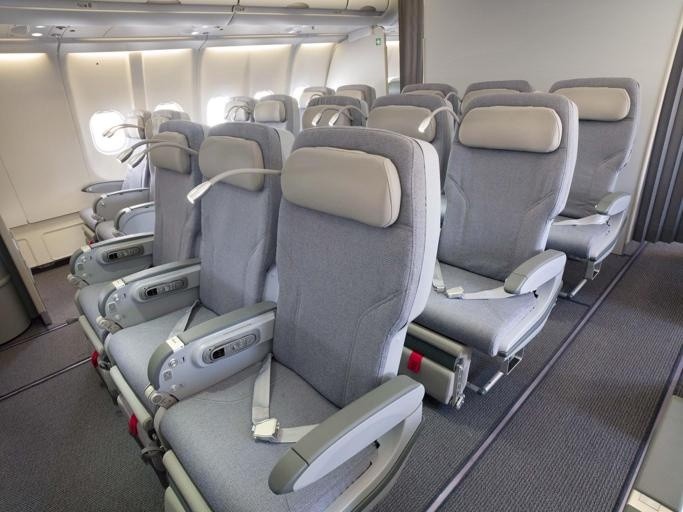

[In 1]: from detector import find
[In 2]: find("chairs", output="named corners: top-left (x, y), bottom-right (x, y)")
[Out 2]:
top-left (220, 77), bottom-right (579, 412)
top-left (68, 107), bottom-right (441, 511)
top-left (544, 77), bottom-right (644, 302)
